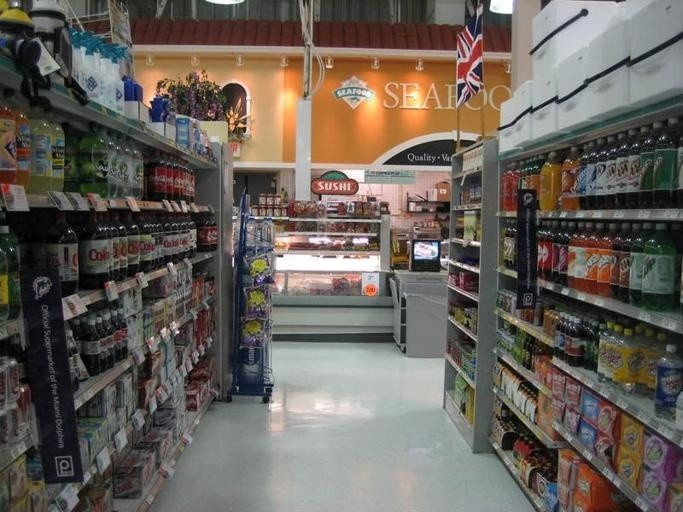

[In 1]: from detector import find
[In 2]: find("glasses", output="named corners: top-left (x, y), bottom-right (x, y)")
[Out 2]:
top-left (141, 52), bottom-right (513, 76)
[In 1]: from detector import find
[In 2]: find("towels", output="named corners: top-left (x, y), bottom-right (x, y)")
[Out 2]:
top-left (403, 279), bottom-right (447, 357)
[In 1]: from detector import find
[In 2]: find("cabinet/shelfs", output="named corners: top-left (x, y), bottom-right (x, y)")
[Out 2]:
top-left (423, 217), bottom-right (439, 228)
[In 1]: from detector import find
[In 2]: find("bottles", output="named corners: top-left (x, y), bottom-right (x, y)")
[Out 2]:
top-left (423, 217), bottom-right (439, 228)
top-left (441, 117), bottom-right (682, 497)
top-left (0, 103), bottom-right (275, 383)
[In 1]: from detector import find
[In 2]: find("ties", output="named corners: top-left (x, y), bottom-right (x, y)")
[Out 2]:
top-left (412, 239), bottom-right (440, 271)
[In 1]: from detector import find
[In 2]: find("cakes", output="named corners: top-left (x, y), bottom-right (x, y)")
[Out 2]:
top-left (0, 355), bottom-right (32, 448)
top-left (496, 286), bottom-right (596, 338)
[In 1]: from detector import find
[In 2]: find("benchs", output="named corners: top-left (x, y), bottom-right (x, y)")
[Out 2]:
top-left (455, 3), bottom-right (485, 107)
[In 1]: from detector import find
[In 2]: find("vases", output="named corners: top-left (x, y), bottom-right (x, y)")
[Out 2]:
top-left (153, 66), bottom-right (227, 124)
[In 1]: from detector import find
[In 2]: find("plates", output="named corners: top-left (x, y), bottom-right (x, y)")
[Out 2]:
top-left (489, 93), bottom-right (683, 511)
top-left (1, 49), bottom-right (221, 512)
top-left (233, 213), bottom-right (381, 298)
top-left (438, 134), bottom-right (496, 455)
